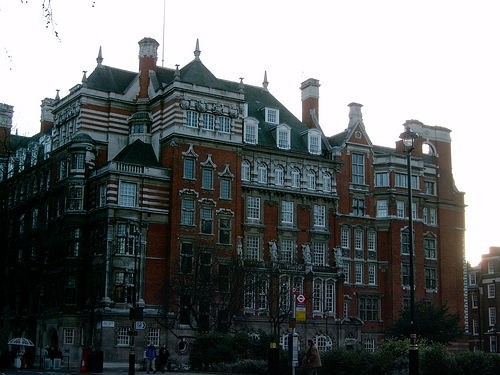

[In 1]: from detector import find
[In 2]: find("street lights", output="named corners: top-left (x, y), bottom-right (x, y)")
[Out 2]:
top-left (399, 127), bottom-right (418, 375)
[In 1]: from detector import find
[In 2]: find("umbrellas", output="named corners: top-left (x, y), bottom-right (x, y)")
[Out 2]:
top-left (7, 336), bottom-right (35, 352)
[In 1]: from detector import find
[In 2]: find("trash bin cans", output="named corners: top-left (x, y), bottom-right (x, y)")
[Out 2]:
top-left (88, 350), bottom-right (104, 373)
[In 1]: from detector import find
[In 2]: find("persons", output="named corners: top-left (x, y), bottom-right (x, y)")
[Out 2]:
top-left (42, 344), bottom-right (63, 360)
top-left (144, 340), bottom-right (157, 374)
top-left (303, 339), bottom-right (322, 375)
top-left (16, 345), bottom-right (26, 370)
top-left (158, 343), bottom-right (170, 374)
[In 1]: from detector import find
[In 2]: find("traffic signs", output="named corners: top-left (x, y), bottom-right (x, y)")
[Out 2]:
top-left (136, 321), bottom-right (147, 330)
